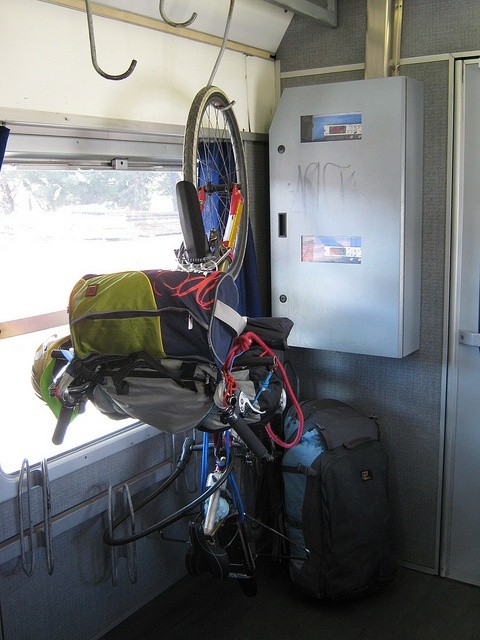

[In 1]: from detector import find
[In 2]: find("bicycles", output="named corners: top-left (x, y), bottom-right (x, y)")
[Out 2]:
top-left (104, 85), bottom-right (311, 595)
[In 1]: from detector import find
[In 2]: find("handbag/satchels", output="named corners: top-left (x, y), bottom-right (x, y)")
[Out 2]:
top-left (68, 270), bottom-right (248, 371)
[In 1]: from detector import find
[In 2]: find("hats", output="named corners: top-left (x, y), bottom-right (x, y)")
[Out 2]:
top-left (30, 332), bottom-right (78, 423)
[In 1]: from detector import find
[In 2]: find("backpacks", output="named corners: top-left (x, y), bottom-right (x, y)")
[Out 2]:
top-left (276, 397), bottom-right (404, 608)
